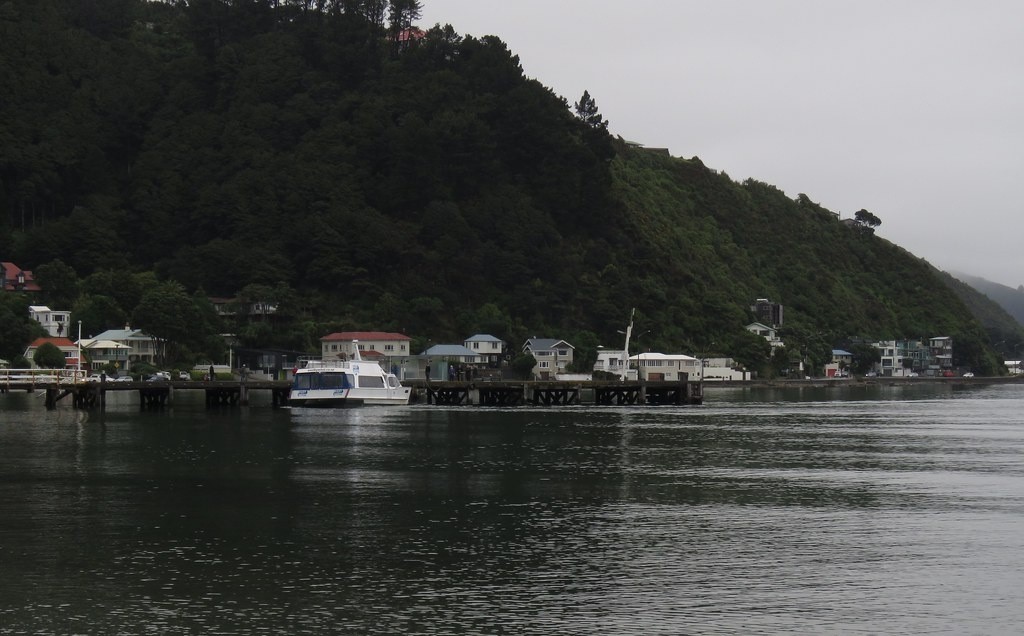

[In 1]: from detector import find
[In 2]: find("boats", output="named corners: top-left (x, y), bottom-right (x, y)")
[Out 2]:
top-left (286, 338), bottom-right (413, 409)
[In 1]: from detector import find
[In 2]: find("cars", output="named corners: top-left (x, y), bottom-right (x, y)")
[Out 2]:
top-left (88, 370), bottom-right (191, 383)
top-left (962, 372), bottom-right (975, 379)
top-left (943, 371), bottom-right (955, 377)
top-left (865, 371), bottom-right (882, 377)
top-left (833, 370), bottom-right (849, 377)
top-left (907, 371), bottom-right (919, 378)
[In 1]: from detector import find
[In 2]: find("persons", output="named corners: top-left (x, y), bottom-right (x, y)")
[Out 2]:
top-left (457, 364), bottom-right (463, 381)
top-left (465, 364), bottom-right (471, 382)
top-left (209, 362), bottom-right (215, 381)
top-left (473, 364), bottom-right (478, 378)
top-left (292, 364), bottom-right (299, 376)
top-left (449, 364), bottom-right (456, 382)
top-left (239, 363), bottom-right (247, 382)
top-left (424, 361), bottom-right (432, 380)
